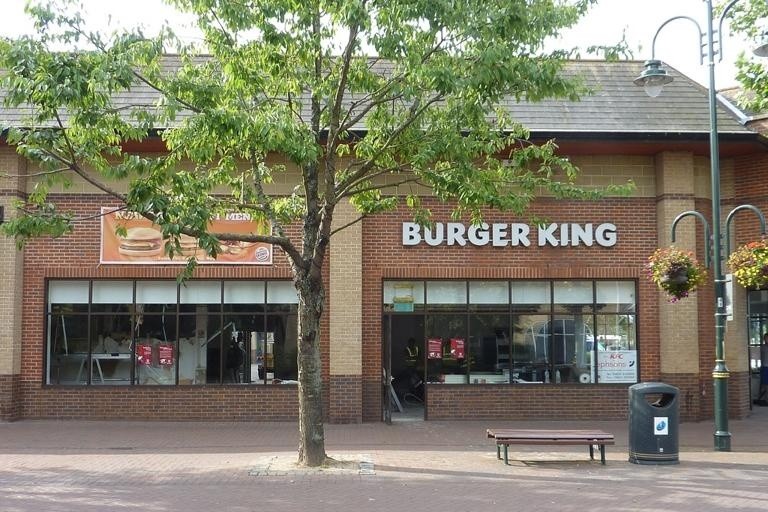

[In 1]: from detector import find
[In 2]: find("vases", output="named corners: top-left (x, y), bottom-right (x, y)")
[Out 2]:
top-left (660, 262), bottom-right (688, 286)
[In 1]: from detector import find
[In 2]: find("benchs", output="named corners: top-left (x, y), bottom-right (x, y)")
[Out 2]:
top-left (485, 429), bottom-right (616, 466)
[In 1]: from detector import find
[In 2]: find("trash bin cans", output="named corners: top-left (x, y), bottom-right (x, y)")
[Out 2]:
top-left (628, 381), bottom-right (680, 465)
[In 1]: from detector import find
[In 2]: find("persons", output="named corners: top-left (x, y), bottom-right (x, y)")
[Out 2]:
top-left (393, 337), bottom-right (420, 381)
top-left (226, 333), bottom-right (244, 383)
top-left (760, 333), bottom-right (768, 385)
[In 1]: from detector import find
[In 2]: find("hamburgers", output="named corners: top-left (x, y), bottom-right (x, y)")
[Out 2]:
top-left (118, 227), bottom-right (162, 256)
top-left (170, 224), bottom-right (202, 256)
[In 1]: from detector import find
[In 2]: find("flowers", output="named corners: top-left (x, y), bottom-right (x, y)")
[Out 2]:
top-left (725, 237), bottom-right (768, 289)
top-left (640, 246), bottom-right (710, 304)
